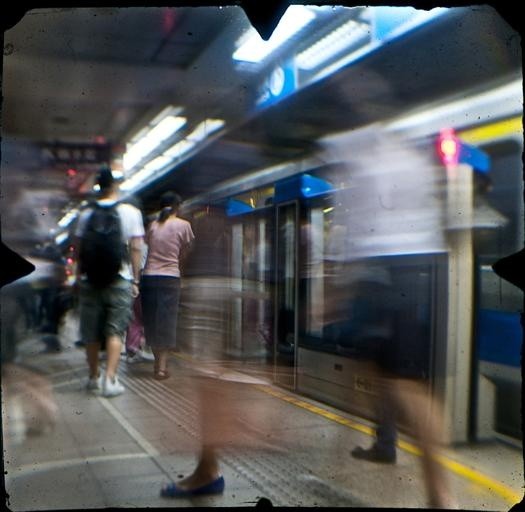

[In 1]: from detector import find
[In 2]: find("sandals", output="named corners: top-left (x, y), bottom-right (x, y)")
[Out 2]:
top-left (155, 367), bottom-right (168, 379)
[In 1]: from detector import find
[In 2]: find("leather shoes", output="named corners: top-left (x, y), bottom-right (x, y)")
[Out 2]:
top-left (162, 476), bottom-right (224, 497)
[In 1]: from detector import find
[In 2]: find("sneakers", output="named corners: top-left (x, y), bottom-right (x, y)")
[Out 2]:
top-left (85, 374), bottom-right (124, 396)
top-left (352, 443), bottom-right (395, 463)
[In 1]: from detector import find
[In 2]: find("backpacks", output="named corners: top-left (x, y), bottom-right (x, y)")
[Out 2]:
top-left (80, 199), bottom-right (125, 289)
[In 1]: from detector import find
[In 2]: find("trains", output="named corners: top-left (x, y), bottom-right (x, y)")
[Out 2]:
top-left (59, 77), bottom-right (523, 458)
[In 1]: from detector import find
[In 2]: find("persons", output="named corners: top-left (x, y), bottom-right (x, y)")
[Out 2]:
top-left (328, 65), bottom-right (460, 510)
top-left (69, 167), bottom-right (146, 398)
top-left (352, 398), bottom-right (398, 465)
top-left (162, 376), bottom-right (226, 499)
top-left (18, 191), bottom-right (228, 379)
